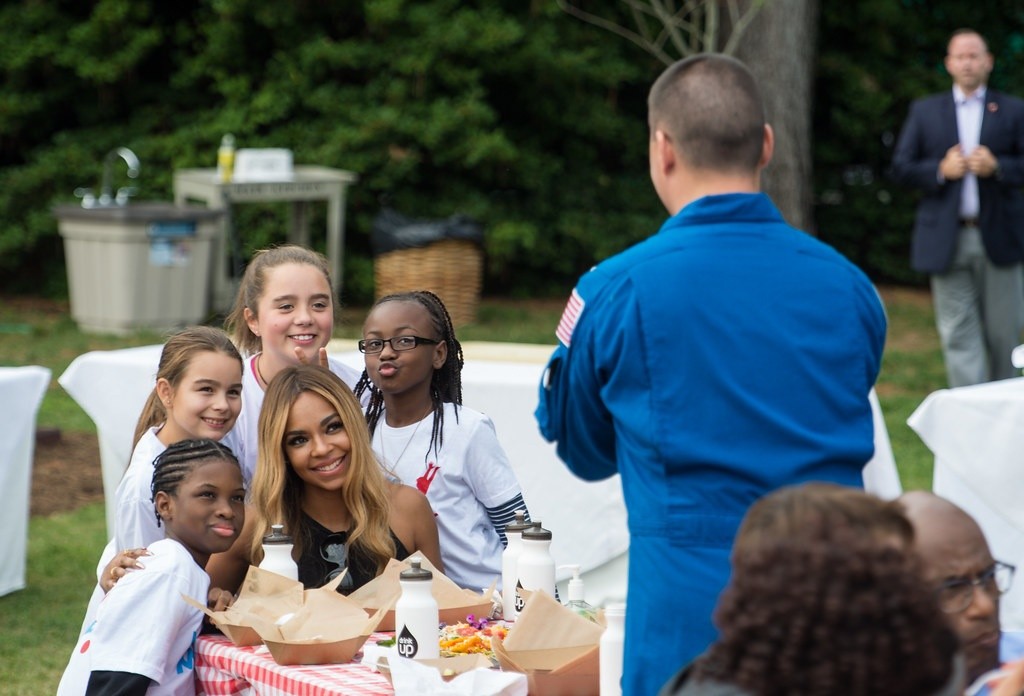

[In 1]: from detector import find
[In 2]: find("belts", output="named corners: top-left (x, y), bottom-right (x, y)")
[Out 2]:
top-left (956, 217), bottom-right (979, 229)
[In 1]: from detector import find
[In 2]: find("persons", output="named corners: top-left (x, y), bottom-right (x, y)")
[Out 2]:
top-left (661, 482), bottom-right (960, 696)
top-left (222, 241), bottom-right (384, 490)
top-left (56, 438), bottom-right (245, 696)
top-left (887, 490), bottom-right (1024, 696)
top-left (294, 290), bottom-right (561, 604)
top-left (534, 54), bottom-right (888, 696)
top-left (892, 28), bottom-right (1024, 388)
top-left (99, 363), bottom-right (446, 623)
top-left (116, 326), bottom-right (252, 554)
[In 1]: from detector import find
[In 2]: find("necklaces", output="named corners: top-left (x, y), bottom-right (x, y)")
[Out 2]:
top-left (257, 354), bottom-right (269, 388)
top-left (380, 403), bottom-right (433, 472)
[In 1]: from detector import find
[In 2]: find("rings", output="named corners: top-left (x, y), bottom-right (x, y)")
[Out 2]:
top-left (111, 567), bottom-right (119, 579)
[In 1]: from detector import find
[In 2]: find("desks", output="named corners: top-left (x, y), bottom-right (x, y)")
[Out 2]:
top-left (908, 375), bottom-right (1024, 641)
top-left (55, 336), bottom-right (904, 607)
top-left (172, 163), bottom-right (355, 319)
top-left (190, 628), bottom-right (506, 696)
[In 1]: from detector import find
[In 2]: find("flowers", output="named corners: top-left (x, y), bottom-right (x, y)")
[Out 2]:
top-left (435, 611), bottom-right (511, 657)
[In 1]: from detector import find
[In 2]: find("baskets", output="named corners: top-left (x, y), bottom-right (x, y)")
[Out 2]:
top-left (373, 235), bottom-right (485, 336)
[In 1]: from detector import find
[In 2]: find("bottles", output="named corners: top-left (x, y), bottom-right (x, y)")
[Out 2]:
top-left (514, 521), bottom-right (557, 621)
top-left (395, 556), bottom-right (440, 660)
top-left (502, 510), bottom-right (533, 622)
top-left (216, 134), bottom-right (234, 182)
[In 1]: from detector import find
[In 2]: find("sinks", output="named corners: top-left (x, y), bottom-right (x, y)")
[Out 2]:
top-left (54, 200), bottom-right (229, 239)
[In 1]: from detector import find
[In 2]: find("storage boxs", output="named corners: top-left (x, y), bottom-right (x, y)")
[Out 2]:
top-left (210, 598), bottom-right (495, 668)
top-left (375, 652), bottom-right (495, 688)
top-left (50, 202), bottom-right (226, 340)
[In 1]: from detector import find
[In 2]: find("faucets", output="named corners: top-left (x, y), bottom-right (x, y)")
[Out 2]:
top-left (73, 145), bottom-right (141, 210)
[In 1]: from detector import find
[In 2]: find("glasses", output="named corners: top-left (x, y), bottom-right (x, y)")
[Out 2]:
top-left (931, 561), bottom-right (1014, 614)
top-left (359, 335), bottom-right (440, 354)
top-left (321, 531), bottom-right (353, 594)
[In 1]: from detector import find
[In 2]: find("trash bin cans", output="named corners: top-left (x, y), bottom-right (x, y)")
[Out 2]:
top-left (373, 217), bottom-right (483, 331)
